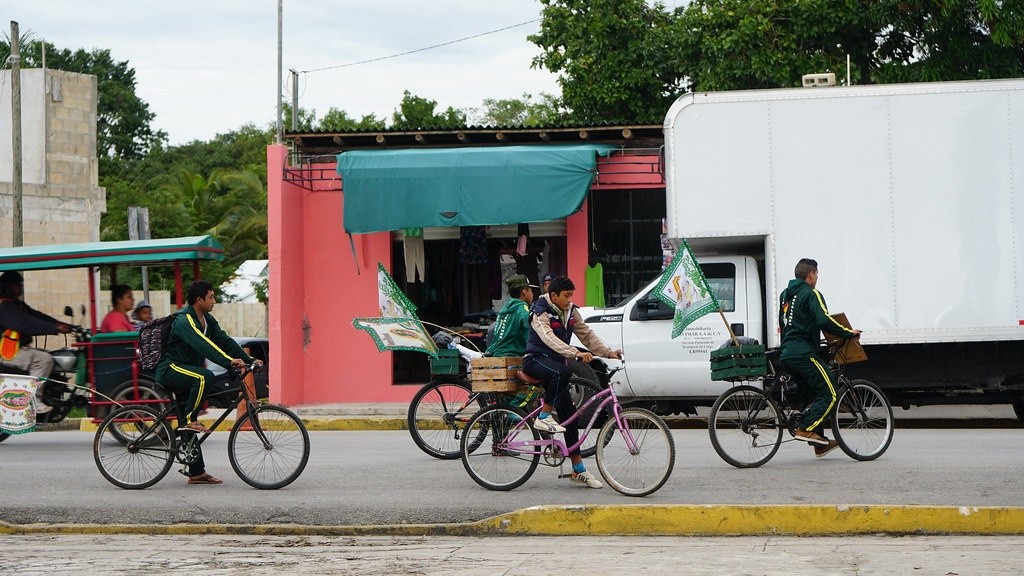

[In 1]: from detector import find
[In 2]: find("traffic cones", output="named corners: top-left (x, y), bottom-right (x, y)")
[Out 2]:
top-left (224, 346), bottom-right (268, 431)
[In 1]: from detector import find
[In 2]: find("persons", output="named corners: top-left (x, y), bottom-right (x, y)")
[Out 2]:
top-left (522, 276), bottom-right (624, 488)
top-left (543, 272), bottom-right (559, 293)
top-left (155, 282), bottom-right (263, 484)
top-left (100, 284), bottom-right (138, 333)
top-left (779, 258), bottom-right (861, 458)
top-left (0, 271), bottom-right (74, 414)
top-left (484, 275), bottom-right (545, 457)
top-left (132, 300), bottom-right (152, 330)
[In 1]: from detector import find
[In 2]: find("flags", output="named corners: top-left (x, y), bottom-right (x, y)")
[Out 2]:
top-left (352, 318), bottom-right (439, 363)
top-left (378, 261), bottom-right (418, 319)
top-left (652, 242), bottom-right (720, 340)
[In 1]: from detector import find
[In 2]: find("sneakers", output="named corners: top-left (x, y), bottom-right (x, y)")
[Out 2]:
top-left (795, 427), bottom-right (829, 445)
top-left (572, 468), bottom-right (602, 488)
top-left (534, 415), bottom-right (566, 432)
top-left (814, 439), bottom-right (839, 457)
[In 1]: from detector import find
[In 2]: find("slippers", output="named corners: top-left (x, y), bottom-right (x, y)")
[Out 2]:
top-left (187, 472), bottom-right (221, 485)
top-left (178, 419), bottom-right (210, 433)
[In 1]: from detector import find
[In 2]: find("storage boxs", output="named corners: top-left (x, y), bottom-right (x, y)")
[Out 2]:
top-left (470, 355), bottom-right (523, 393)
top-left (710, 345), bottom-right (768, 381)
top-left (429, 348), bottom-right (460, 376)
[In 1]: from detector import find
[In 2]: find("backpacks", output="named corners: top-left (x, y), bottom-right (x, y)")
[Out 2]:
top-left (138, 311), bottom-right (177, 371)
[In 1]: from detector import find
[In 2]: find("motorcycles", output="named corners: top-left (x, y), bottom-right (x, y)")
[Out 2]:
top-left (0, 233), bottom-right (225, 449)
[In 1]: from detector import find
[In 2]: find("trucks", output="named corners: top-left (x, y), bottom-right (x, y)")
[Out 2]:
top-left (548, 76), bottom-right (1024, 428)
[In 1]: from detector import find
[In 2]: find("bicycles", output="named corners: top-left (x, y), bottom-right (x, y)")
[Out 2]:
top-left (93, 363), bottom-right (310, 490)
top-left (408, 346), bottom-right (619, 461)
top-left (457, 353), bottom-right (678, 498)
top-left (706, 329), bottom-right (895, 470)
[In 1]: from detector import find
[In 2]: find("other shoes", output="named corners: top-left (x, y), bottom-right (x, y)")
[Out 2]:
top-left (35, 397), bottom-right (54, 414)
top-left (492, 444), bottom-right (521, 456)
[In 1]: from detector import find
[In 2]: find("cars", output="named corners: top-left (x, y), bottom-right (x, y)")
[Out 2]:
top-left (205, 334), bottom-right (269, 409)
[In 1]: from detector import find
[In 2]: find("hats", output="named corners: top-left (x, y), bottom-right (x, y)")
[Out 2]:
top-left (132, 302), bottom-right (154, 320)
top-left (505, 274), bottom-right (540, 289)
top-left (543, 273), bottom-right (557, 281)
